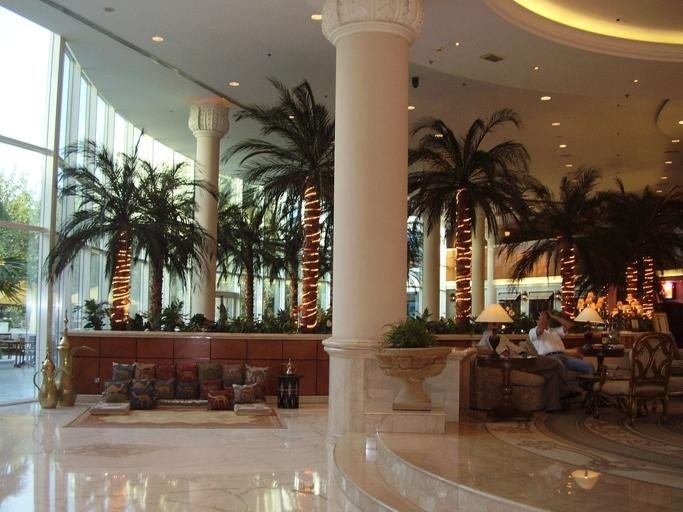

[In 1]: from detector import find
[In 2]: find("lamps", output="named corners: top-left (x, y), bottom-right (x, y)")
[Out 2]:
top-left (573, 308), bottom-right (605, 352)
top-left (577, 291), bottom-right (643, 317)
top-left (474, 304), bottom-right (514, 358)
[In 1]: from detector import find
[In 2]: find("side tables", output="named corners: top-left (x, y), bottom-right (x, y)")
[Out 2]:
top-left (477, 358), bottom-right (536, 421)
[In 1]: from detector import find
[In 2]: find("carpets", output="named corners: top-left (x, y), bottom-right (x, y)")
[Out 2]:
top-left (61, 403), bottom-right (290, 429)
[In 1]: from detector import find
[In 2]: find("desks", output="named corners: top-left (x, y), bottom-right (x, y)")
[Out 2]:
top-left (275, 374), bottom-right (304, 409)
top-left (578, 348), bottom-right (624, 412)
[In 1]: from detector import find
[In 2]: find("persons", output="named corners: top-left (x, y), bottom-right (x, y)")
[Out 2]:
top-left (476, 322), bottom-right (583, 414)
top-left (527, 308), bottom-right (613, 408)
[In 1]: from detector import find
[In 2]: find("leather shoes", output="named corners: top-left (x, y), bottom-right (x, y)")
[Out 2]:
top-left (559, 389), bottom-right (582, 400)
top-left (546, 408), bottom-right (569, 414)
top-left (600, 400), bottom-right (617, 408)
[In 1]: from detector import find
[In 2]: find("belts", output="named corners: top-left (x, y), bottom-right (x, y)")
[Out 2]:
top-left (545, 351), bottom-right (564, 356)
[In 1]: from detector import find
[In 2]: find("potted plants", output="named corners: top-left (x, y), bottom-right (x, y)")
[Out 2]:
top-left (375, 306), bottom-right (451, 410)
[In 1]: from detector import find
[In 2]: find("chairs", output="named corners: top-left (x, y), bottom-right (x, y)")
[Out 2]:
top-left (0, 333), bottom-right (36, 368)
top-left (591, 332), bottom-right (674, 427)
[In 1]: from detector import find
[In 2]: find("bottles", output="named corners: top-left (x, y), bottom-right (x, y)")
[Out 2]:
top-left (56, 310), bottom-right (76, 407)
top-left (32, 352), bottom-right (58, 408)
top-left (601, 325), bottom-right (609, 348)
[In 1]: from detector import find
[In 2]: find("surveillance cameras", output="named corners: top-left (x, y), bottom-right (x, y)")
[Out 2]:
top-left (412, 77), bottom-right (419, 88)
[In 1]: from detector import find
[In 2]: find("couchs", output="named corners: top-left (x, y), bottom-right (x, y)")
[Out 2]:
top-left (477, 345), bottom-right (632, 412)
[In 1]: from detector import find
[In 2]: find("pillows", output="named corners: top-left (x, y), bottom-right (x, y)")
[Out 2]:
top-left (105, 360), bottom-right (269, 410)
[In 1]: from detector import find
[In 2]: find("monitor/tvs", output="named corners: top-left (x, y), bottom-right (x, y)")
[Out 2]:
top-left (659, 281), bottom-right (676, 300)
